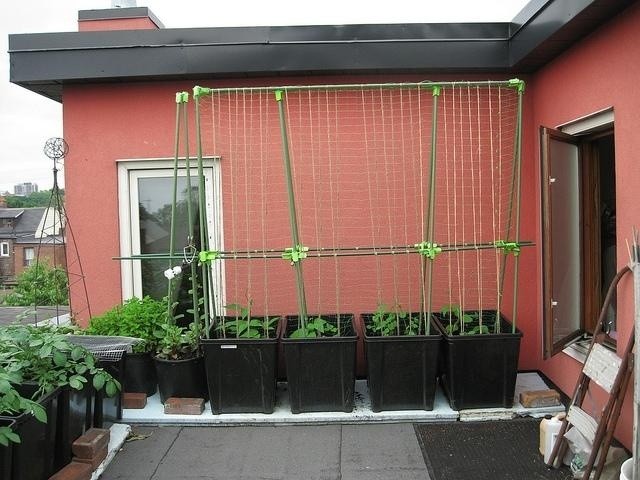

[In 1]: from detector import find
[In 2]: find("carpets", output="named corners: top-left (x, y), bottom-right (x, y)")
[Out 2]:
top-left (414, 417), bottom-right (584, 480)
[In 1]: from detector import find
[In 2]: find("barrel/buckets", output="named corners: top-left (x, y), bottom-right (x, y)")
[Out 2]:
top-left (617, 458), bottom-right (633, 479)
top-left (539, 410), bottom-right (566, 465)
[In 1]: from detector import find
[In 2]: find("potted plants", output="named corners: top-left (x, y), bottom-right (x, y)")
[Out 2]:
top-left (279, 313), bottom-right (360, 415)
top-left (431, 303), bottom-right (524, 411)
top-left (81, 295), bottom-right (167, 397)
top-left (1, 319), bottom-right (118, 480)
top-left (198, 292), bottom-right (283, 415)
top-left (151, 296), bottom-right (207, 406)
top-left (358, 299), bottom-right (444, 414)
